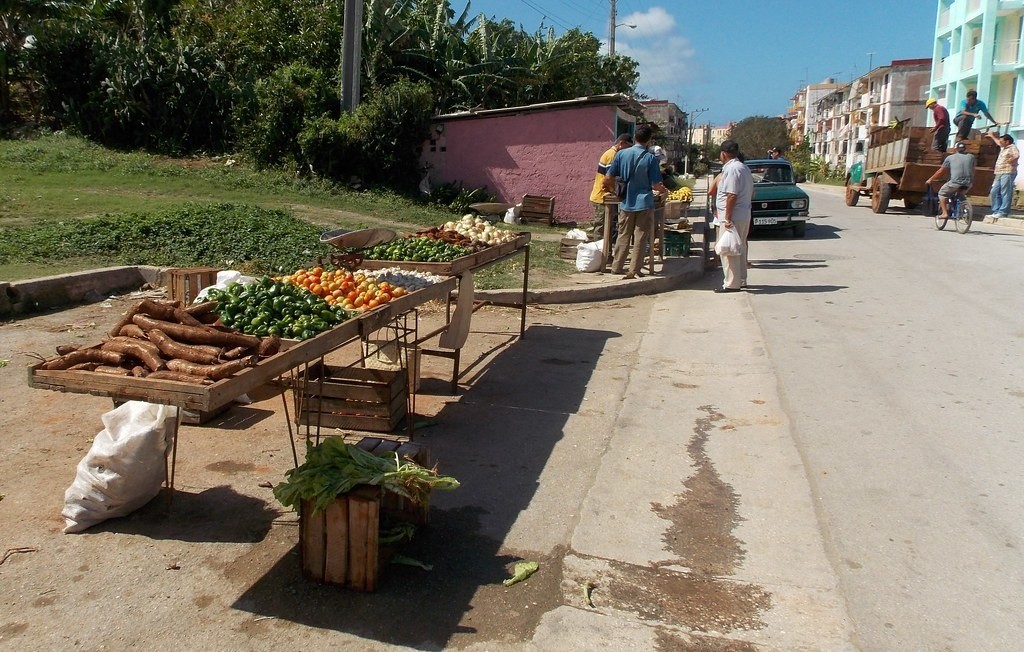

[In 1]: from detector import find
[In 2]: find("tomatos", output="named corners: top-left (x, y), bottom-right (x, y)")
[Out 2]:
top-left (270, 267), bottom-right (407, 310)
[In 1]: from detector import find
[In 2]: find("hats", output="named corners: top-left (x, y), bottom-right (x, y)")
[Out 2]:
top-left (955, 141), bottom-right (965, 148)
top-left (618, 134), bottom-right (634, 146)
top-left (769, 146), bottom-right (781, 154)
top-left (649, 146), bottom-right (664, 157)
top-left (720, 140), bottom-right (739, 154)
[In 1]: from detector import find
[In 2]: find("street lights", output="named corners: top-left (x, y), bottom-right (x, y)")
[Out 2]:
top-left (609, 23), bottom-right (637, 54)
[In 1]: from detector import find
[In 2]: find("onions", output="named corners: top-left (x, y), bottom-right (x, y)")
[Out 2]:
top-left (444, 214), bottom-right (517, 244)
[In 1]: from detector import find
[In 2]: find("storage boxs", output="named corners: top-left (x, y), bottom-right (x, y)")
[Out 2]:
top-left (520, 194), bottom-right (556, 225)
top-left (292, 362), bottom-right (408, 431)
top-left (298, 438), bottom-right (428, 589)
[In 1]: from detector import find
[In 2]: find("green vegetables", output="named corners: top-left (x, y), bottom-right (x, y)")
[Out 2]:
top-left (273, 437), bottom-right (459, 571)
top-left (502, 562), bottom-right (538, 585)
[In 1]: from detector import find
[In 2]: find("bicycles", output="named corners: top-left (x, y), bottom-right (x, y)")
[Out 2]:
top-left (930, 179), bottom-right (973, 234)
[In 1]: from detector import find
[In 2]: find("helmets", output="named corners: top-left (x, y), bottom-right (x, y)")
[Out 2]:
top-left (925, 99), bottom-right (936, 109)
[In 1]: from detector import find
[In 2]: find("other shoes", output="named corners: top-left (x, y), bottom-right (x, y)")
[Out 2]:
top-left (985, 213), bottom-right (1008, 219)
top-left (606, 255), bottom-right (614, 264)
top-left (611, 269), bottom-right (627, 274)
top-left (632, 270), bottom-right (645, 277)
top-left (713, 286), bottom-right (748, 293)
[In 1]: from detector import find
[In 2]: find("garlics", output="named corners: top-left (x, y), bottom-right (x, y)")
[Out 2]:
top-left (352, 267), bottom-right (442, 291)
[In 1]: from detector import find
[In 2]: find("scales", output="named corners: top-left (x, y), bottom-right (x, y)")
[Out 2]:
top-left (469, 202), bottom-right (511, 225)
top-left (316, 229), bottom-right (397, 272)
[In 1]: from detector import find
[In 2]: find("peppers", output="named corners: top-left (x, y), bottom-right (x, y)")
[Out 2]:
top-left (361, 237), bottom-right (473, 262)
top-left (197, 275), bottom-right (360, 340)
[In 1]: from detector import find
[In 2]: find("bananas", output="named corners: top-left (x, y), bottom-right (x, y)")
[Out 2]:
top-left (669, 186), bottom-right (693, 200)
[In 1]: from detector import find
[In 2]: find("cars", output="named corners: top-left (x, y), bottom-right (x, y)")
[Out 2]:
top-left (744, 159), bottom-right (811, 238)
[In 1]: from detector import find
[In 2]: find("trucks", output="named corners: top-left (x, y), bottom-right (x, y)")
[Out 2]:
top-left (845, 124), bottom-right (1001, 213)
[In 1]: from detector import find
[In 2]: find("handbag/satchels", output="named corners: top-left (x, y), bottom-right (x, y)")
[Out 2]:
top-left (362, 321), bottom-right (422, 393)
top-left (714, 217), bottom-right (744, 256)
top-left (614, 176), bottom-right (627, 199)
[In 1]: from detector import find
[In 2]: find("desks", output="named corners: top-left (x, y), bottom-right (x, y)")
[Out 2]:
top-left (26, 229), bottom-right (530, 497)
top-left (604, 194), bottom-right (667, 274)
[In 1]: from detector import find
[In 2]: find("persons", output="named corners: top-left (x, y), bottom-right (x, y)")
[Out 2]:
top-left (953, 91), bottom-right (1000, 148)
top-left (924, 141), bottom-right (977, 219)
top-left (924, 97), bottom-right (950, 153)
top-left (707, 139), bottom-right (754, 293)
top-left (762, 146), bottom-right (791, 182)
top-left (589, 123), bottom-right (677, 280)
top-left (984, 131), bottom-right (1020, 218)
